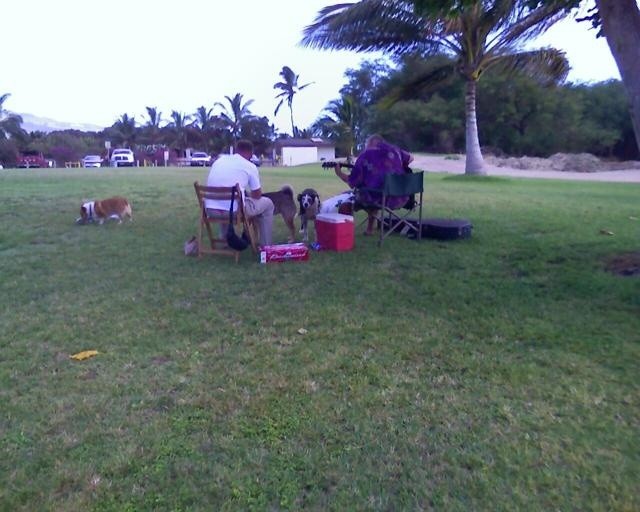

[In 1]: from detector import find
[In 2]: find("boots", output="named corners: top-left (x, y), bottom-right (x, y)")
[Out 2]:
top-left (112, 149), bottom-right (135, 166)
top-left (15, 146), bottom-right (45, 168)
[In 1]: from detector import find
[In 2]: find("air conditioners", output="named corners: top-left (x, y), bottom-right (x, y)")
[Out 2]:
top-left (297, 187), bottom-right (322, 243)
top-left (79, 197), bottom-right (134, 225)
top-left (243, 183), bottom-right (297, 245)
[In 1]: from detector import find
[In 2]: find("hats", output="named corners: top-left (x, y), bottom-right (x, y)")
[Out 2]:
top-left (82, 156), bottom-right (104, 169)
top-left (191, 151), bottom-right (211, 166)
top-left (250, 154), bottom-right (260, 166)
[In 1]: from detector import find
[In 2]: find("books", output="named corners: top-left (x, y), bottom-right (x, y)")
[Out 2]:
top-left (315, 213), bottom-right (354, 251)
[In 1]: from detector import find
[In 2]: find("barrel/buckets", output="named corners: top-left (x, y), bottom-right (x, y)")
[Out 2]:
top-left (362, 230), bottom-right (372, 236)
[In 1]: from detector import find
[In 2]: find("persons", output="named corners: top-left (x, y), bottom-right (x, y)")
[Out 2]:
top-left (204, 140), bottom-right (275, 247)
top-left (334, 132), bottom-right (415, 236)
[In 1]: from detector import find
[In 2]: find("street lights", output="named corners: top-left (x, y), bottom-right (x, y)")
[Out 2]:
top-left (228, 223), bottom-right (250, 251)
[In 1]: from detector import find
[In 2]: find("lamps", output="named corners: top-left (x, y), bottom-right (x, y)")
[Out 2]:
top-left (350, 170), bottom-right (425, 248)
top-left (194, 181), bottom-right (259, 263)
